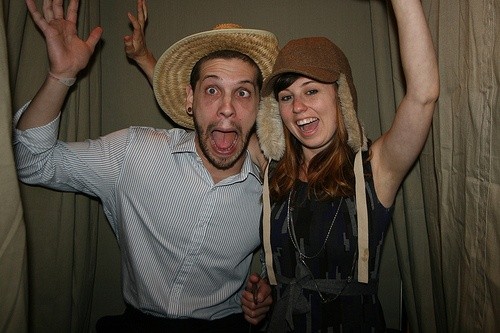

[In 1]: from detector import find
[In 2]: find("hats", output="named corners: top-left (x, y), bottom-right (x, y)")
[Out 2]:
top-left (153, 24), bottom-right (280, 130)
top-left (256, 37), bottom-right (368, 285)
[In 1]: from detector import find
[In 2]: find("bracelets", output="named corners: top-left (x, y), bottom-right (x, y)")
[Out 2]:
top-left (48, 70), bottom-right (77, 86)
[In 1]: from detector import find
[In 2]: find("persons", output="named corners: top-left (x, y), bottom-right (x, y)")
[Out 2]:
top-left (124, 0), bottom-right (440, 333)
top-left (11, 0), bottom-right (281, 333)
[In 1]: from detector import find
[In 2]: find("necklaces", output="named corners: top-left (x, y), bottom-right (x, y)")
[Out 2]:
top-left (288, 182), bottom-right (343, 257)
